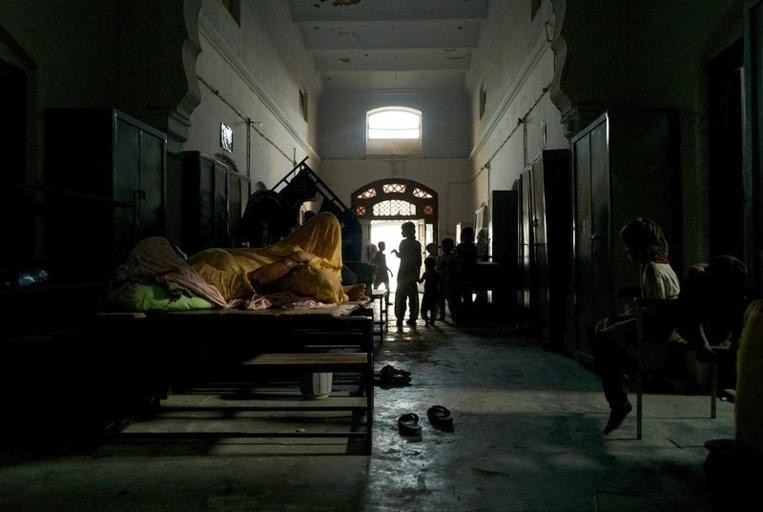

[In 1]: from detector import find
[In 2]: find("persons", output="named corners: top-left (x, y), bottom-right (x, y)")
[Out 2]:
top-left (454, 225), bottom-right (478, 326)
top-left (436, 237), bottom-right (456, 322)
top-left (372, 241), bottom-right (393, 305)
top-left (417, 256), bottom-right (440, 326)
top-left (672, 255), bottom-right (753, 354)
top-left (585, 215), bottom-right (681, 441)
top-left (426, 242), bottom-right (443, 319)
top-left (110, 208), bottom-right (372, 313)
top-left (390, 222), bottom-right (422, 327)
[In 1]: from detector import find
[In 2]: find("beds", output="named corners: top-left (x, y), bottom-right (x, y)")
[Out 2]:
top-left (94, 273), bottom-right (390, 456)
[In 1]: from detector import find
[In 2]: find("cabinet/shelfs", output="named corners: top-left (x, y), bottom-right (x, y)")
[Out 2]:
top-left (0, 282), bottom-right (76, 463)
top-left (569, 107), bottom-right (682, 367)
top-left (517, 148), bottom-right (572, 348)
top-left (181, 151), bottom-right (251, 248)
top-left (487, 190), bottom-right (518, 335)
top-left (41, 104), bottom-right (168, 278)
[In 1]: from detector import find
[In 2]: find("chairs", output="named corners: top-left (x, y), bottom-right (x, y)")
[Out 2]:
top-left (591, 254), bottom-right (748, 439)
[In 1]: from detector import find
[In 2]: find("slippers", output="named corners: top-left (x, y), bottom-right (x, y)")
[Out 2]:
top-left (426, 405), bottom-right (453, 426)
top-left (373, 365), bottom-right (411, 386)
top-left (397, 412), bottom-right (421, 436)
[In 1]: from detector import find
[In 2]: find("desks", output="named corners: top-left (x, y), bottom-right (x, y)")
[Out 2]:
top-left (474, 261), bottom-right (502, 336)
